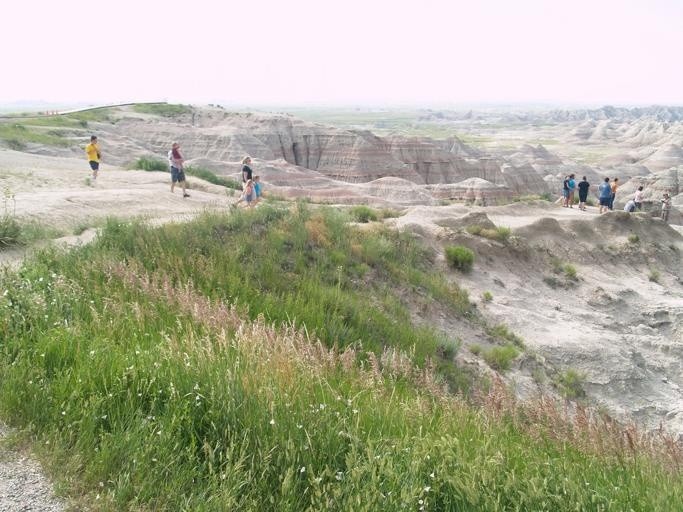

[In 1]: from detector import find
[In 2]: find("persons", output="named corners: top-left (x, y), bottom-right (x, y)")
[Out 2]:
top-left (251, 174), bottom-right (261, 203)
top-left (597, 176), bottom-right (612, 215)
top-left (561, 174), bottom-right (572, 207)
top-left (239, 178), bottom-right (254, 209)
top-left (230, 155), bottom-right (256, 212)
top-left (566, 173), bottom-right (575, 208)
top-left (631, 185), bottom-right (645, 211)
top-left (576, 175), bottom-right (589, 211)
top-left (84, 134), bottom-right (101, 188)
top-left (608, 177), bottom-right (618, 212)
top-left (659, 192), bottom-right (671, 222)
top-left (166, 141), bottom-right (190, 197)
top-left (171, 142), bottom-right (183, 175)
top-left (622, 197), bottom-right (636, 213)
